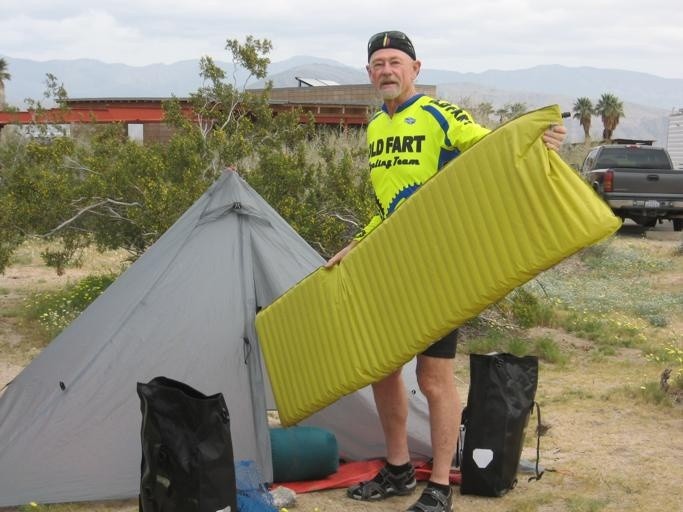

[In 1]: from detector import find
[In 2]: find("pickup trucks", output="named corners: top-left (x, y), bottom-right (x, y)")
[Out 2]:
top-left (579, 145), bottom-right (683, 233)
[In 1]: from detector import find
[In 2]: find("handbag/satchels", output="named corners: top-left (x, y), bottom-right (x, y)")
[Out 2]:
top-left (136, 377), bottom-right (236, 511)
top-left (460, 352), bottom-right (538, 496)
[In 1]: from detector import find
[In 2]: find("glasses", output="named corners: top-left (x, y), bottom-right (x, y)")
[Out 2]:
top-left (367, 31), bottom-right (415, 47)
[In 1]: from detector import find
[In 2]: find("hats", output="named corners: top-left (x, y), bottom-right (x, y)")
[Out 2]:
top-left (368, 33), bottom-right (416, 61)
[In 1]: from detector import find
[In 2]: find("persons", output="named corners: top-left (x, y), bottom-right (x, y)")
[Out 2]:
top-left (320, 27), bottom-right (570, 508)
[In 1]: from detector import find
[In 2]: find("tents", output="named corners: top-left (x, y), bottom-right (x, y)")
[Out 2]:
top-left (2, 162), bottom-right (548, 510)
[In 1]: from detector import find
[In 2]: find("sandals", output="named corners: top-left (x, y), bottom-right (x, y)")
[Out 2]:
top-left (347, 460), bottom-right (416, 501)
top-left (405, 488), bottom-right (453, 512)
